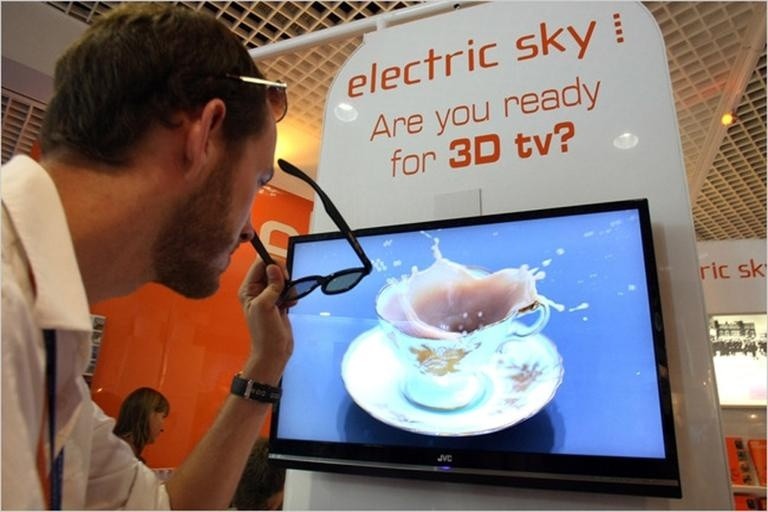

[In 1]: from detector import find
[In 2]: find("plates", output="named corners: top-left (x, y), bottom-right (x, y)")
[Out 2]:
top-left (340, 323), bottom-right (568, 441)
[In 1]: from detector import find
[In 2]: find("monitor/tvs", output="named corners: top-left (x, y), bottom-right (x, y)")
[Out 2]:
top-left (265, 198), bottom-right (682, 499)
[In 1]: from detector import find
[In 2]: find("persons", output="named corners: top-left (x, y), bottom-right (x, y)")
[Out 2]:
top-left (1, 2), bottom-right (298, 511)
top-left (113, 388), bottom-right (169, 464)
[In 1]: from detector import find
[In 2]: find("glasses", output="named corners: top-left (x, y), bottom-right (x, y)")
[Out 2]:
top-left (220, 72), bottom-right (287, 124)
top-left (250, 159), bottom-right (372, 301)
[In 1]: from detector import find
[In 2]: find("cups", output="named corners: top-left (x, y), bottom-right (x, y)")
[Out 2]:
top-left (374, 262), bottom-right (548, 409)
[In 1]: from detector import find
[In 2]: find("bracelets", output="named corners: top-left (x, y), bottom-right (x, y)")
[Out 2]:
top-left (231, 371), bottom-right (282, 404)
